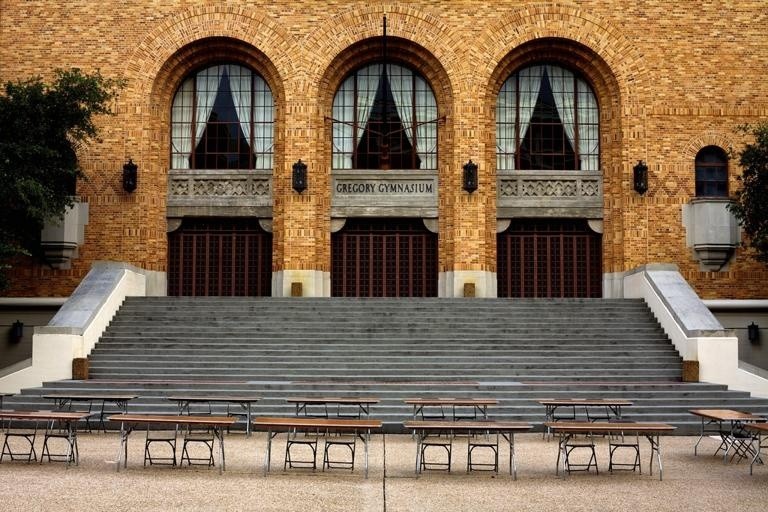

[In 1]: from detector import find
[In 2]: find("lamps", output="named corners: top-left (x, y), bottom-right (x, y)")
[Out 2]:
top-left (630, 157), bottom-right (648, 195)
top-left (9, 319), bottom-right (26, 342)
top-left (463, 159), bottom-right (478, 197)
top-left (122, 158), bottom-right (139, 193)
top-left (290, 157), bottom-right (311, 198)
top-left (747, 320), bottom-right (761, 348)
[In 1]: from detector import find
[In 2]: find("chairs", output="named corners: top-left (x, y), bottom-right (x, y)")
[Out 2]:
top-left (43, 392), bottom-right (138, 440)
top-left (168, 394), bottom-right (262, 439)
top-left (106, 411), bottom-right (238, 474)
top-left (537, 395), bottom-right (634, 443)
top-left (248, 412), bottom-right (383, 478)
top-left (283, 394), bottom-right (382, 443)
top-left (400, 395), bottom-right (502, 442)
top-left (691, 407), bottom-right (768, 477)
top-left (403, 419), bottom-right (535, 480)
top-left (3, 408), bottom-right (95, 471)
top-left (544, 420), bottom-right (680, 480)
